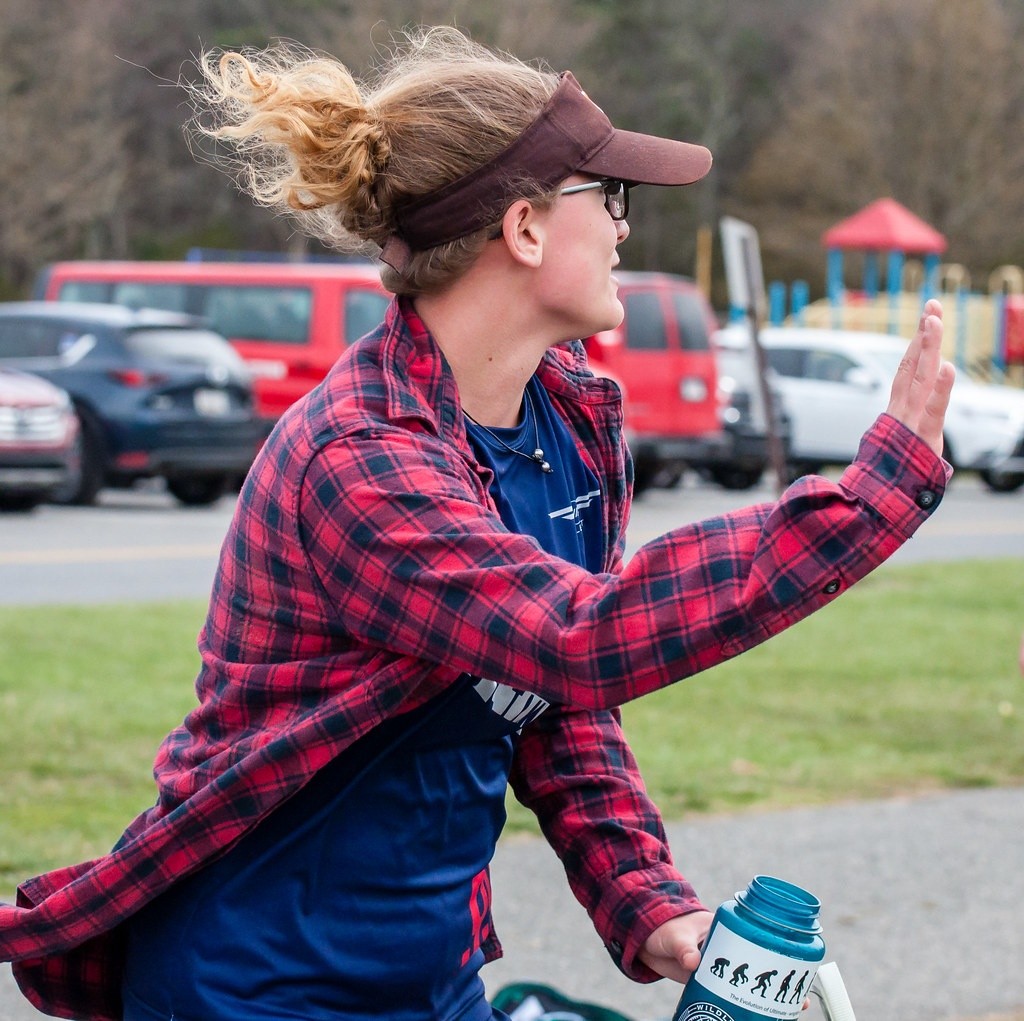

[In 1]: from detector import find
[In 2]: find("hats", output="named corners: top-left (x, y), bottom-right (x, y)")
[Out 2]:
top-left (371, 71), bottom-right (713, 274)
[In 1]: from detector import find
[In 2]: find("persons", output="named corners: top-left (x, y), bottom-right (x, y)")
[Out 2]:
top-left (0, 26), bottom-right (956, 1021)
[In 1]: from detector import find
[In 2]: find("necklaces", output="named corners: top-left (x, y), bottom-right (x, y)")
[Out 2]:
top-left (461, 385), bottom-right (550, 474)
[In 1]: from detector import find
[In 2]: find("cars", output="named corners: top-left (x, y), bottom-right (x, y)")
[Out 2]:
top-left (0, 367), bottom-right (81, 510)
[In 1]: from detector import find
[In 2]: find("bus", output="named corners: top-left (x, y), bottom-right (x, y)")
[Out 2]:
top-left (33, 258), bottom-right (772, 501)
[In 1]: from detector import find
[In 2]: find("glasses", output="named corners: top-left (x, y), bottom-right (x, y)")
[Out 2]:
top-left (489, 177), bottom-right (629, 240)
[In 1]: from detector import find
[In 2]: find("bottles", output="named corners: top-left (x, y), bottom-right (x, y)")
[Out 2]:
top-left (672, 876), bottom-right (826, 1021)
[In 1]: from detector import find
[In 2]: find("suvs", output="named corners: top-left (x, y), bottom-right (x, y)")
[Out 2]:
top-left (684, 325), bottom-right (1023, 496)
top-left (0, 300), bottom-right (276, 511)
